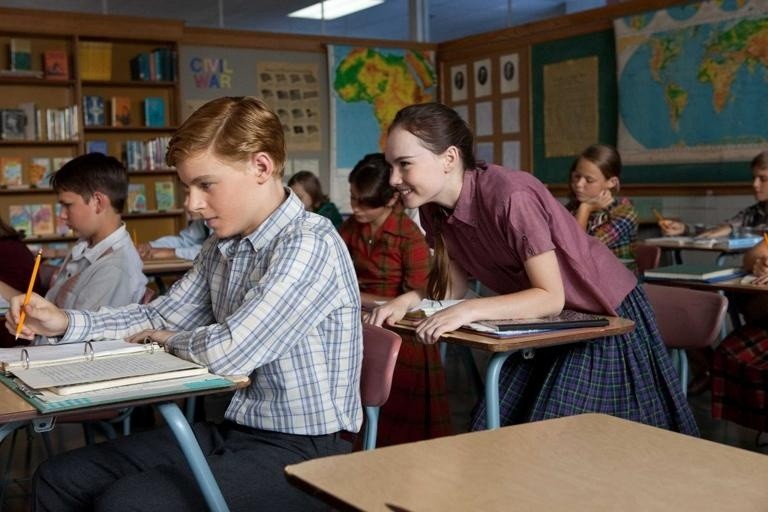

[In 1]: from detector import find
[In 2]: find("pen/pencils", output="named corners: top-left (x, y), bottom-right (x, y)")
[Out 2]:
top-left (132, 229), bottom-right (137, 247)
top-left (15, 248), bottom-right (43, 340)
top-left (651, 208), bottom-right (668, 227)
top-left (763, 232), bottom-right (768, 247)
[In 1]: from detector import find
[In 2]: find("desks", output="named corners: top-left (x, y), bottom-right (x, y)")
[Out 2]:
top-left (0, 338), bottom-right (252, 508)
top-left (284, 412), bottom-right (767, 510)
top-left (0, 282), bottom-right (24, 318)
top-left (645, 267), bottom-right (766, 327)
top-left (362, 308), bottom-right (636, 447)
top-left (640, 236), bottom-right (764, 266)
top-left (140, 257), bottom-right (193, 272)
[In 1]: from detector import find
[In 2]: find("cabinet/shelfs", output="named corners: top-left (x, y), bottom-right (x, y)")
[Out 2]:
top-left (0, 6), bottom-right (186, 244)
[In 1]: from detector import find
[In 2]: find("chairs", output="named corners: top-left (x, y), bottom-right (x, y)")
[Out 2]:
top-left (632, 243), bottom-right (660, 272)
top-left (640, 284), bottom-right (727, 397)
top-left (361, 323), bottom-right (402, 449)
